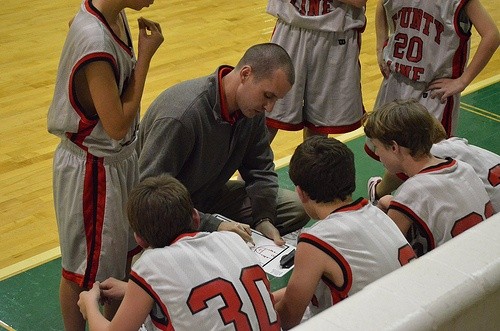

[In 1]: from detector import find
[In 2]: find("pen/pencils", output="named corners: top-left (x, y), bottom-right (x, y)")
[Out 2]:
top-left (238, 223), bottom-right (255, 245)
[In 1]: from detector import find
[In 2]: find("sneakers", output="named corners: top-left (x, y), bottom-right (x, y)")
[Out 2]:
top-left (367, 176), bottom-right (383, 205)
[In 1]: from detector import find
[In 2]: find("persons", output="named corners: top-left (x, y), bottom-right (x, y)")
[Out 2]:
top-left (48, 0), bottom-right (164, 331)
top-left (373, 1), bottom-right (499, 152)
top-left (422, 116), bottom-right (500, 210)
top-left (136, 43), bottom-right (312, 244)
top-left (253, 1), bottom-right (369, 149)
top-left (367, 98), bottom-right (495, 248)
top-left (275, 136), bottom-right (423, 328)
top-left (79, 176), bottom-right (280, 329)
